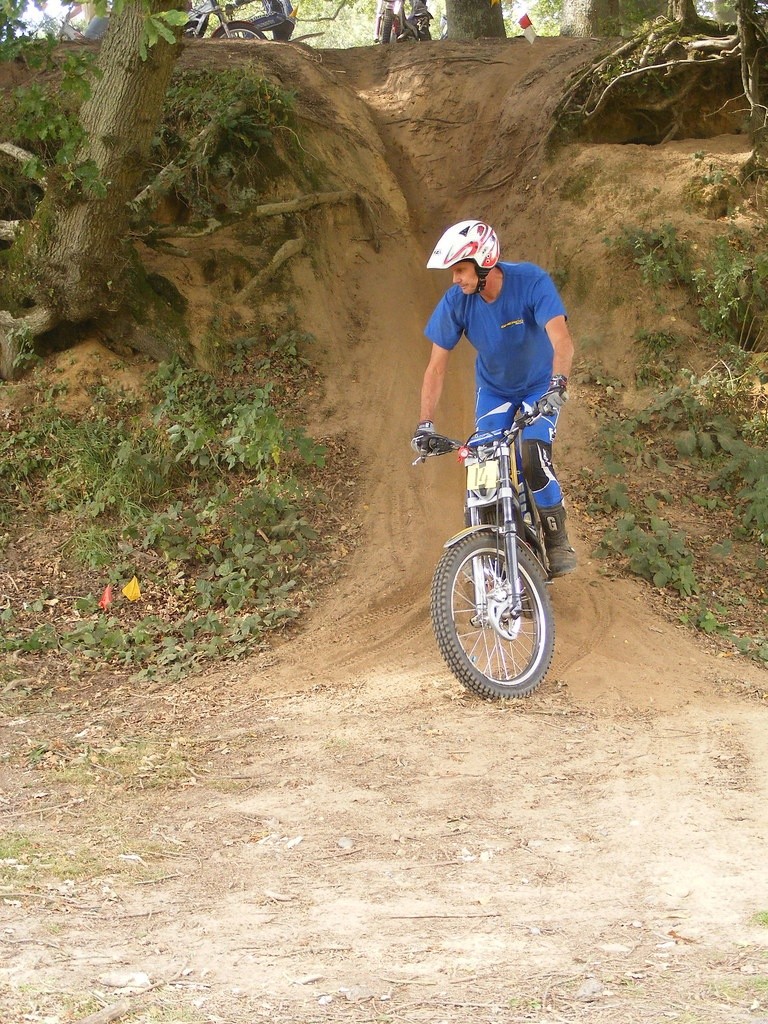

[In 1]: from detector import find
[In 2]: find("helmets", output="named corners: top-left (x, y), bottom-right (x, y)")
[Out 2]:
top-left (427, 219), bottom-right (500, 269)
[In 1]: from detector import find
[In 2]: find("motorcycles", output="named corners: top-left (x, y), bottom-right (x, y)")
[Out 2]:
top-left (182, 0), bottom-right (269, 41)
top-left (374, 0), bottom-right (430, 44)
top-left (56, 5), bottom-right (86, 39)
top-left (225, 4), bottom-right (325, 50)
top-left (412, 390), bottom-right (571, 699)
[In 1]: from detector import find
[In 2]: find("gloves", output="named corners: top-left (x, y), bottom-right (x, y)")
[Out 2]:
top-left (411, 421), bottom-right (435, 454)
top-left (538, 388), bottom-right (569, 416)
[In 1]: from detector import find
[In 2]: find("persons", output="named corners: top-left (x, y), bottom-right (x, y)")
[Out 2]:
top-left (411, 220), bottom-right (575, 577)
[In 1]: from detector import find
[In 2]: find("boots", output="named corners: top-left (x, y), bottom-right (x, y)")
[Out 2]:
top-left (539, 505), bottom-right (576, 576)
